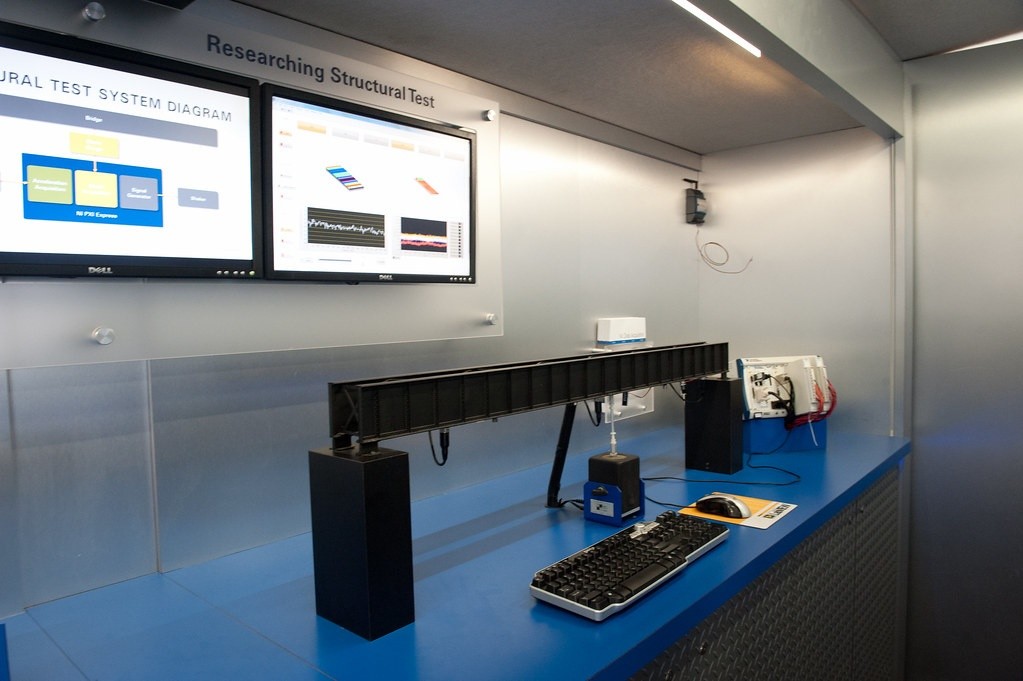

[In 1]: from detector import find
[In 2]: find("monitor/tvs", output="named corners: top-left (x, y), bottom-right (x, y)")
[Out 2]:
top-left (0, 18), bottom-right (479, 285)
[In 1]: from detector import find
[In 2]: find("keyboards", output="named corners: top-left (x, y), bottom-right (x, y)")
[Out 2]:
top-left (528, 510), bottom-right (729, 622)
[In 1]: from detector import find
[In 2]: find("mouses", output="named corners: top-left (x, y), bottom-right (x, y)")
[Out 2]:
top-left (696, 494), bottom-right (752, 518)
